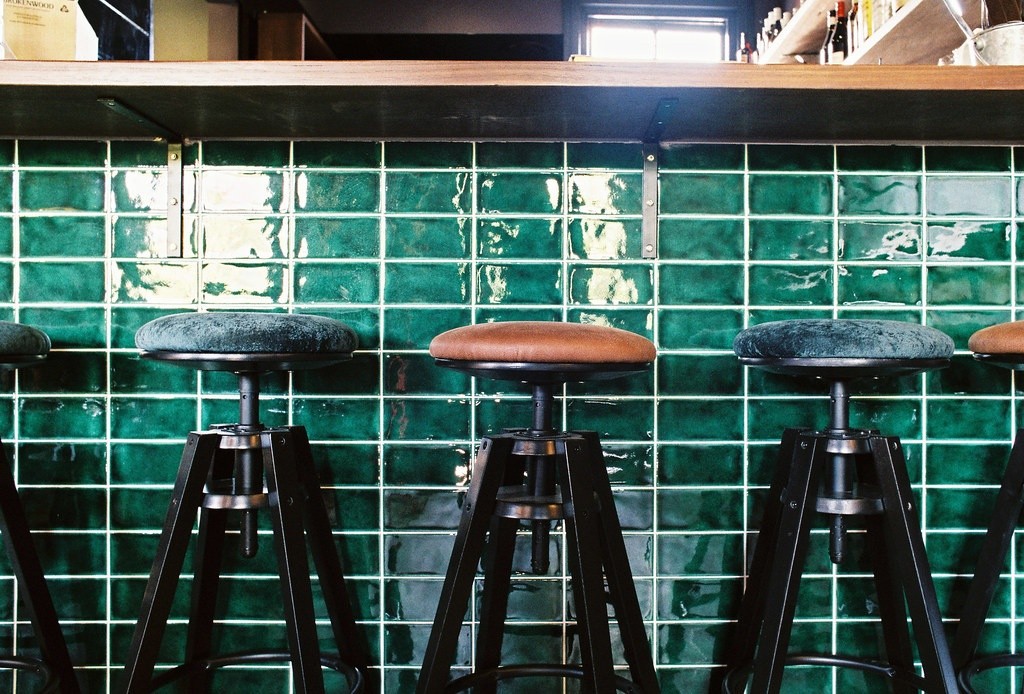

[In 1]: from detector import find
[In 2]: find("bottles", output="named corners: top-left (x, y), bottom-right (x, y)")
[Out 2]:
top-left (736, 7), bottom-right (798, 63)
top-left (847, 0), bottom-right (908, 54)
top-left (819, 0), bottom-right (837, 64)
top-left (831, 0), bottom-right (848, 64)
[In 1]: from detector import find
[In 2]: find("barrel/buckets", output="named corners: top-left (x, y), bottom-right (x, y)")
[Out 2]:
top-left (969, 22), bottom-right (1024, 65)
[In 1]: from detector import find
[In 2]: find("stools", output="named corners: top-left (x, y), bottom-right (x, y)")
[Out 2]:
top-left (950, 320), bottom-right (1024, 694)
top-left (118, 312), bottom-right (377, 694)
top-left (0, 319), bottom-right (82, 694)
top-left (716, 319), bottom-right (970, 694)
top-left (413, 322), bottom-right (662, 694)
